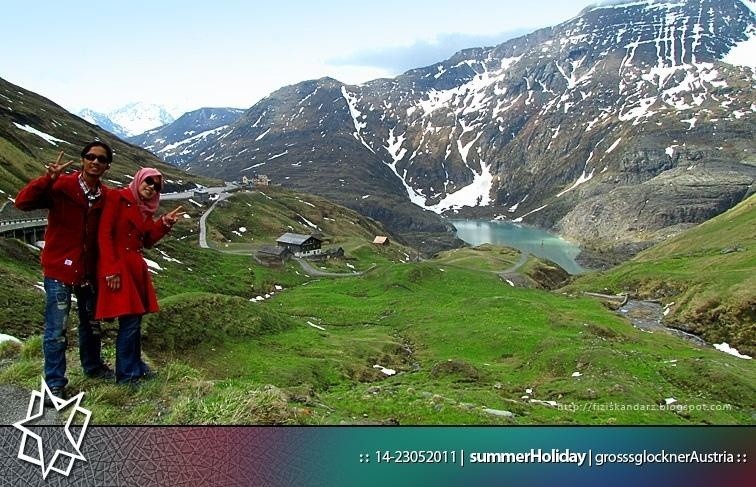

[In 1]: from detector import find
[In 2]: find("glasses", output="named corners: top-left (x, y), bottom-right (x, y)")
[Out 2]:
top-left (145, 176), bottom-right (161, 191)
top-left (84, 153), bottom-right (108, 164)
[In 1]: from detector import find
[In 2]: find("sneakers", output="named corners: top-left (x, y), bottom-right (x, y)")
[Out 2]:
top-left (45, 386), bottom-right (64, 408)
top-left (90, 368), bottom-right (115, 380)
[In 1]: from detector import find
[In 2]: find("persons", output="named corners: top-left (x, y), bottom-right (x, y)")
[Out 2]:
top-left (16, 141), bottom-right (117, 408)
top-left (92, 166), bottom-right (187, 385)
